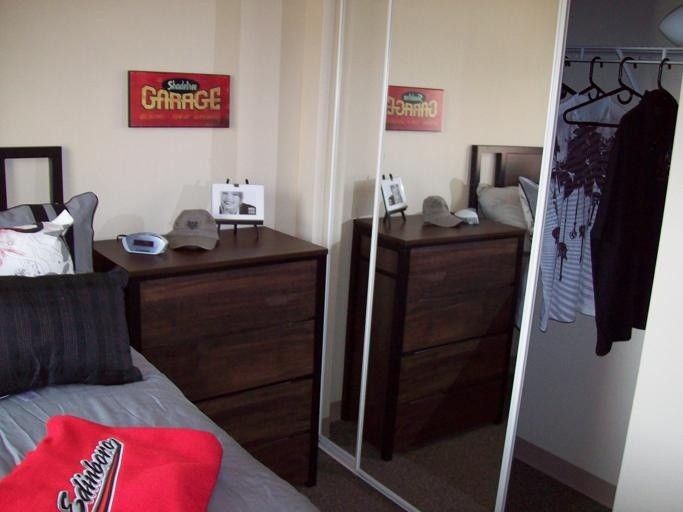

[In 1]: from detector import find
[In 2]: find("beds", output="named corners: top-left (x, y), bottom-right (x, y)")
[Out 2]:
top-left (467, 143), bottom-right (543, 238)
top-left (0, 147), bottom-right (324, 512)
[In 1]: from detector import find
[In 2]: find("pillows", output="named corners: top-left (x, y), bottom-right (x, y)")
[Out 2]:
top-left (0, 416), bottom-right (223, 512)
top-left (476, 176), bottom-right (540, 235)
top-left (1, 191), bottom-right (146, 399)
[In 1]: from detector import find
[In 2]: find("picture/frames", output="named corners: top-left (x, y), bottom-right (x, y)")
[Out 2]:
top-left (210, 183), bottom-right (265, 225)
top-left (378, 178), bottom-right (407, 215)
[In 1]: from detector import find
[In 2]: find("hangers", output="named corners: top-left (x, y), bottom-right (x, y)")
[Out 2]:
top-left (561, 54), bottom-right (681, 133)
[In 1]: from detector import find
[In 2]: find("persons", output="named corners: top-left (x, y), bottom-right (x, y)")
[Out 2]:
top-left (387, 185), bottom-right (403, 206)
top-left (220, 191), bottom-right (256, 214)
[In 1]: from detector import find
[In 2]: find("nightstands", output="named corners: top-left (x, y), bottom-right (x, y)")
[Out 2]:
top-left (91, 226), bottom-right (328, 487)
top-left (339, 209), bottom-right (523, 464)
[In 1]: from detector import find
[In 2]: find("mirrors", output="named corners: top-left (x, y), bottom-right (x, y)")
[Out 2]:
top-left (318, 0), bottom-right (572, 512)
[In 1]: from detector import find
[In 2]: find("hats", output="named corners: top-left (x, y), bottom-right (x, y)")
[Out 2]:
top-left (423, 195), bottom-right (462, 228)
top-left (170, 209), bottom-right (219, 250)
